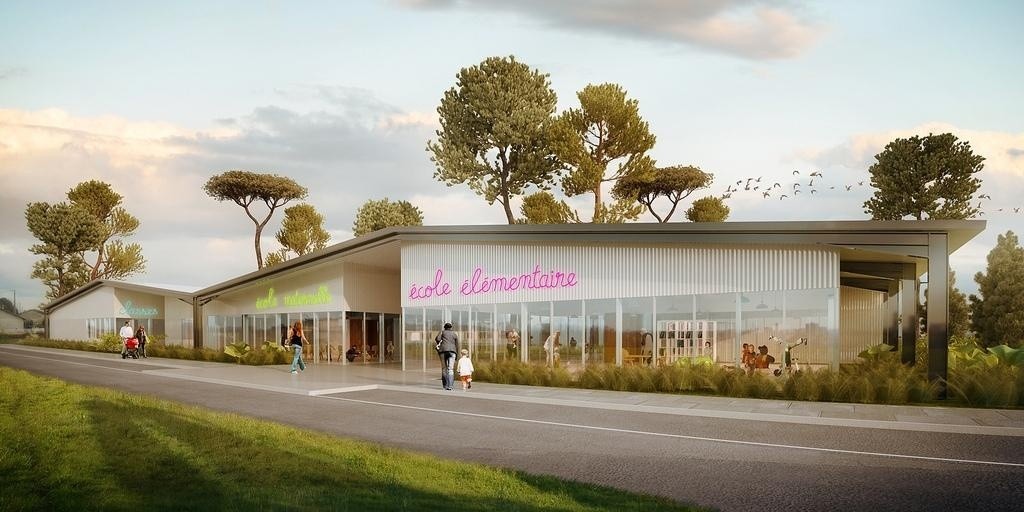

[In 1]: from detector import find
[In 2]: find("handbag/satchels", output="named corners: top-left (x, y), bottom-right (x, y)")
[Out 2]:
top-left (433, 340), bottom-right (442, 350)
top-left (145, 337), bottom-right (150, 343)
top-left (288, 337), bottom-right (292, 347)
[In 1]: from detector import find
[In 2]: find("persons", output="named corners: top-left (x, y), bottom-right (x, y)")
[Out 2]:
top-left (312, 340), bottom-right (395, 362)
top-left (550, 346), bottom-right (562, 369)
top-left (118, 320), bottom-right (135, 358)
top-left (434, 321), bottom-right (459, 392)
top-left (346, 343), bottom-right (362, 363)
top-left (510, 328), bottom-right (519, 349)
top-left (568, 335), bottom-right (578, 353)
top-left (287, 318), bottom-right (310, 374)
top-left (504, 324), bottom-right (519, 360)
top-left (455, 347), bottom-right (474, 391)
top-left (738, 342), bottom-right (775, 375)
top-left (131, 323), bottom-right (150, 358)
top-left (637, 326), bottom-right (652, 364)
top-left (541, 330), bottom-right (560, 369)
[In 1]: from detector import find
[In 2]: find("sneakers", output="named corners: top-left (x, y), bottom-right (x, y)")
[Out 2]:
top-left (292, 371), bottom-right (298, 374)
